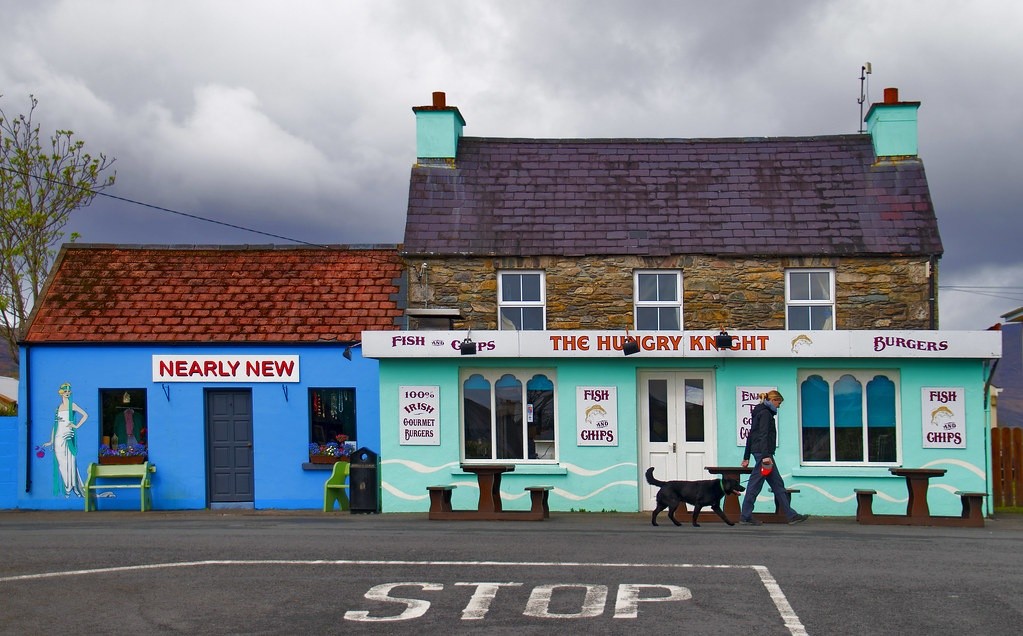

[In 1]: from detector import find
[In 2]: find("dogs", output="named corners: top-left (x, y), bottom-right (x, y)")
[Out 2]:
top-left (646, 466), bottom-right (746, 527)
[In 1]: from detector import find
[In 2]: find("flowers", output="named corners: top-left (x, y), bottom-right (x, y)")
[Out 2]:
top-left (99, 443), bottom-right (150, 457)
top-left (310, 434), bottom-right (355, 457)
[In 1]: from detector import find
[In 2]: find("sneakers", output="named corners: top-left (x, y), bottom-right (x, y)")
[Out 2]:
top-left (789, 514), bottom-right (811, 524)
top-left (739, 518), bottom-right (762, 525)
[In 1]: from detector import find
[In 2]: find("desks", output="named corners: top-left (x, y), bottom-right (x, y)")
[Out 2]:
top-left (704, 466), bottom-right (755, 523)
top-left (463, 466), bottom-right (516, 520)
top-left (888, 467), bottom-right (947, 526)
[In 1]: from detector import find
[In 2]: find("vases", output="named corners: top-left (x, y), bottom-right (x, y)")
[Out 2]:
top-left (308, 453), bottom-right (349, 464)
top-left (100, 455), bottom-right (145, 464)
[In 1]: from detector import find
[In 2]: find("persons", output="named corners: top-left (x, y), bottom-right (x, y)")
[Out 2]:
top-left (739, 389), bottom-right (810, 526)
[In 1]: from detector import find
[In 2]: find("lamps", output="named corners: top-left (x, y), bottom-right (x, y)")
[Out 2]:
top-left (622, 325), bottom-right (641, 356)
top-left (716, 325), bottom-right (732, 348)
top-left (343, 341), bottom-right (362, 362)
top-left (459, 325), bottom-right (477, 355)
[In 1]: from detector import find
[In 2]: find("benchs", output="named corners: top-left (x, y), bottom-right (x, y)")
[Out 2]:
top-left (955, 490), bottom-right (990, 527)
top-left (525, 486), bottom-right (555, 520)
top-left (426, 485), bottom-right (458, 520)
top-left (81, 462), bottom-right (156, 512)
top-left (768, 487), bottom-right (800, 525)
top-left (854, 488), bottom-right (878, 525)
top-left (324, 446), bottom-right (382, 513)
